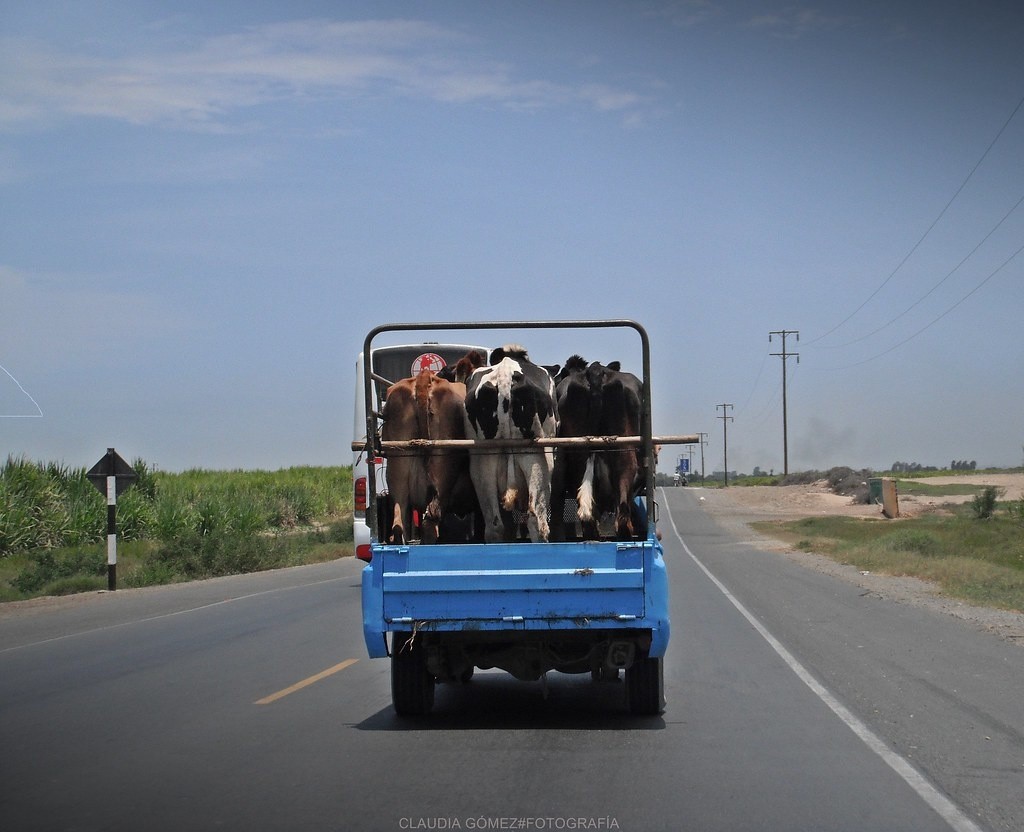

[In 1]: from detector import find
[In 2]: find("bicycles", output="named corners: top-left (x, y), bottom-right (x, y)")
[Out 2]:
top-left (674, 480), bottom-right (677, 487)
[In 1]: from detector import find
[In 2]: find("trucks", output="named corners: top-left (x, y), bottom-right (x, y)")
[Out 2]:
top-left (352, 316), bottom-right (698, 718)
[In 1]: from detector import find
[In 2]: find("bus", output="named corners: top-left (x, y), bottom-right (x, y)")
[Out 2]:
top-left (353, 343), bottom-right (492, 563)
